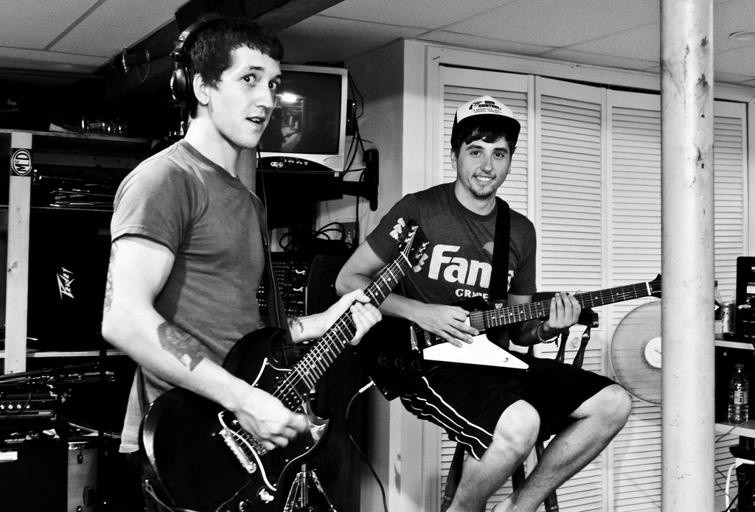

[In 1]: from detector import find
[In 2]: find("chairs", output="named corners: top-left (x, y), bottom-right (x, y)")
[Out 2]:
top-left (441, 291), bottom-right (601, 511)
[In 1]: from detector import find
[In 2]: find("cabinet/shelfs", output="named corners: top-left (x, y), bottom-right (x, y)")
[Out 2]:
top-left (715, 332), bottom-right (755, 436)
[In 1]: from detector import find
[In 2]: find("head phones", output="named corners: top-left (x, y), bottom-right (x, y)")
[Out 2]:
top-left (169, 14), bottom-right (234, 108)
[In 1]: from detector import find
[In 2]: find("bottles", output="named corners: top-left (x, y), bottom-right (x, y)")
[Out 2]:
top-left (714, 277), bottom-right (723, 334)
top-left (725, 363), bottom-right (748, 423)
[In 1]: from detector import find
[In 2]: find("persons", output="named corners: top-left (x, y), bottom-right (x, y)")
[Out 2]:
top-left (334, 95), bottom-right (632, 512)
top-left (102, 19), bottom-right (383, 512)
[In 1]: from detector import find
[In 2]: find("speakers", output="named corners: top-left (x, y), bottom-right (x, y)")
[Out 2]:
top-left (0, 206), bottom-right (120, 353)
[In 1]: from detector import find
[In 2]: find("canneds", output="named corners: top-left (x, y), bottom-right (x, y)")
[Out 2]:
top-left (721, 302), bottom-right (735, 333)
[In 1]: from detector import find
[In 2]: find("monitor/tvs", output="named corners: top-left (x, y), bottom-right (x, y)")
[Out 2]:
top-left (257, 64), bottom-right (348, 172)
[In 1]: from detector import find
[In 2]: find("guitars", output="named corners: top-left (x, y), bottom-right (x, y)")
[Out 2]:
top-left (138, 217), bottom-right (432, 512)
top-left (349, 273), bottom-right (663, 402)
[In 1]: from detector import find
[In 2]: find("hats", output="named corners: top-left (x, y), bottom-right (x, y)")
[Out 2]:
top-left (452, 95), bottom-right (520, 142)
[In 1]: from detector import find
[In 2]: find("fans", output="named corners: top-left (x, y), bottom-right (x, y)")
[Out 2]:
top-left (609, 301), bottom-right (662, 403)
